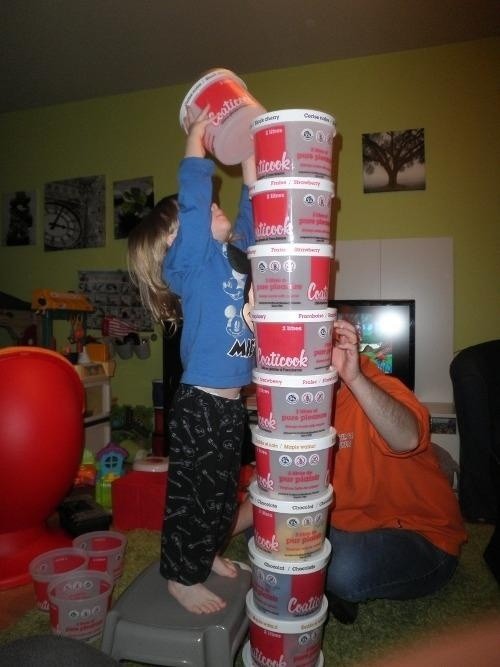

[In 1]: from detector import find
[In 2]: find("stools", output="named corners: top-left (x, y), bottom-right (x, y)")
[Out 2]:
top-left (100, 544), bottom-right (256, 665)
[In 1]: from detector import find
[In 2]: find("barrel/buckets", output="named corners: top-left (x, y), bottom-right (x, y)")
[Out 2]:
top-left (248, 536), bottom-right (332, 621)
top-left (251, 426), bottom-right (337, 502)
top-left (47, 570), bottom-right (114, 645)
top-left (249, 308), bottom-right (338, 374)
top-left (251, 108), bottom-right (337, 181)
top-left (179, 67), bottom-right (268, 167)
top-left (251, 368), bottom-right (338, 440)
top-left (246, 587), bottom-right (329, 666)
top-left (74, 530), bottom-right (127, 581)
top-left (250, 480), bottom-right (334, 561)
top-left (29, 547), bottom-right (88, 611)
top-left (246, 243), bottom-right (333, 309)
top-left (247, 176), bottom-right (335, 245)
top-left (242, 639), bottom-right (324, 667)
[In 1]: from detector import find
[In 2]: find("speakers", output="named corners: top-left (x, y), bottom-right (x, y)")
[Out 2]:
top-left (163, 321), bottom-right (183, 458)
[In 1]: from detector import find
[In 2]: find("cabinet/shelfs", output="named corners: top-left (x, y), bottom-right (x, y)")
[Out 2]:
top-left (80, 372), bottom-right (112, 456)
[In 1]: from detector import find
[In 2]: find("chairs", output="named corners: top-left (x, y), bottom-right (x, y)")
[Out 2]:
top-left (446, 335), bottom-right (499, 528)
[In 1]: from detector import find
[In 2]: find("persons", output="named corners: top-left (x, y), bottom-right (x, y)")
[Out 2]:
top-left (230, 318), bottom-right (468, 626)
top-left (126, 102), bottom-right (257, 615)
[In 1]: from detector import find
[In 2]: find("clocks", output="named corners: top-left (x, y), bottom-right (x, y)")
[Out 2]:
top-left (42, 199), bottom-right (84, 251)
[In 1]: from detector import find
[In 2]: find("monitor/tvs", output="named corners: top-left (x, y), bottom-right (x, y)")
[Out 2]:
top-left (327, 300), bottom-right (415, 393)
